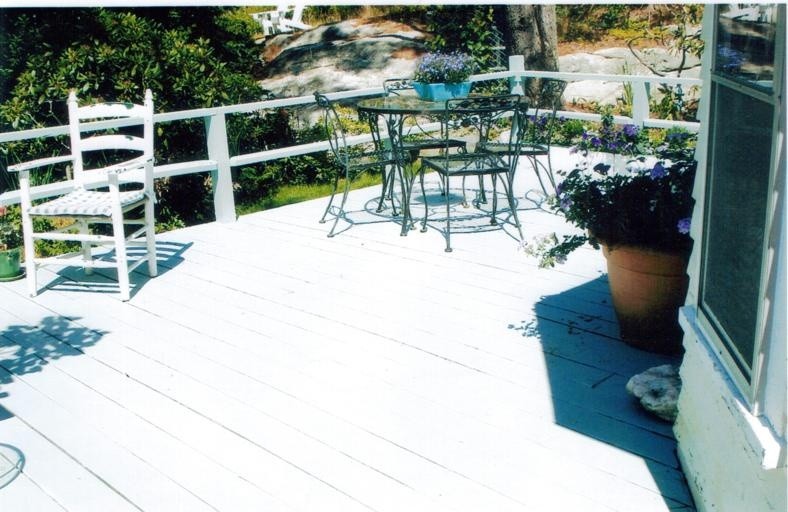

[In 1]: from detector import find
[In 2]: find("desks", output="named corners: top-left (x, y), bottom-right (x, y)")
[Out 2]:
top-left (358, 90), bottom-right (532, 228)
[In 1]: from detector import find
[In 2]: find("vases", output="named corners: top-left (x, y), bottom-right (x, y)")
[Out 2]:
top-left (0, 249), bottom-right (26, 282)
top-left (600, 241), bottom-right (689, 351)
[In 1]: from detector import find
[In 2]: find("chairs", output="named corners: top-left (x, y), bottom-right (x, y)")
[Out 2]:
top-left (7, 89), bottom-right (158, 302)
top-left (384, 81), bottom-right (474, 215)
top-left (476, 78), bottom-right (566, 211)
top-left (417, 92), bottom-right (529, 252)
top-left (314, 90), bottom-right (418, 240)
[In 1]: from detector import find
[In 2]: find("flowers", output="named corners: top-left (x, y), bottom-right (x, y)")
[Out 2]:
top-left (0, 206), bottom-right (23, 248)
top-left (714, 44), bottom-right (746, 77)
top-left (410, 49), bottom-right (479, 84)
top-left (515, 108), bottom-right (699, 271)
